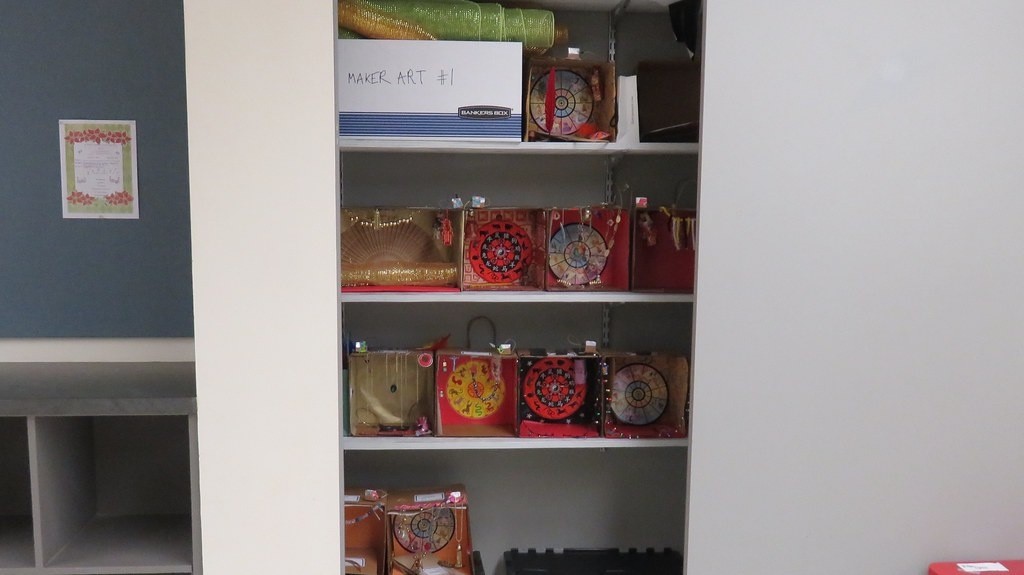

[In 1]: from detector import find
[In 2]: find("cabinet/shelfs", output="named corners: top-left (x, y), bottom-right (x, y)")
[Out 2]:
top-left (333, 0), bottom-right (709, 575)
top-left (0, 397), bottom-right (202, 575)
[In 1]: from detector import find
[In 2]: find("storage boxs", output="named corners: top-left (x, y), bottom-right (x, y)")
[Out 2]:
top-left (503, 546), bottom-right (684, 575)
top-left (337, 37), bottom-right (523, 143)
